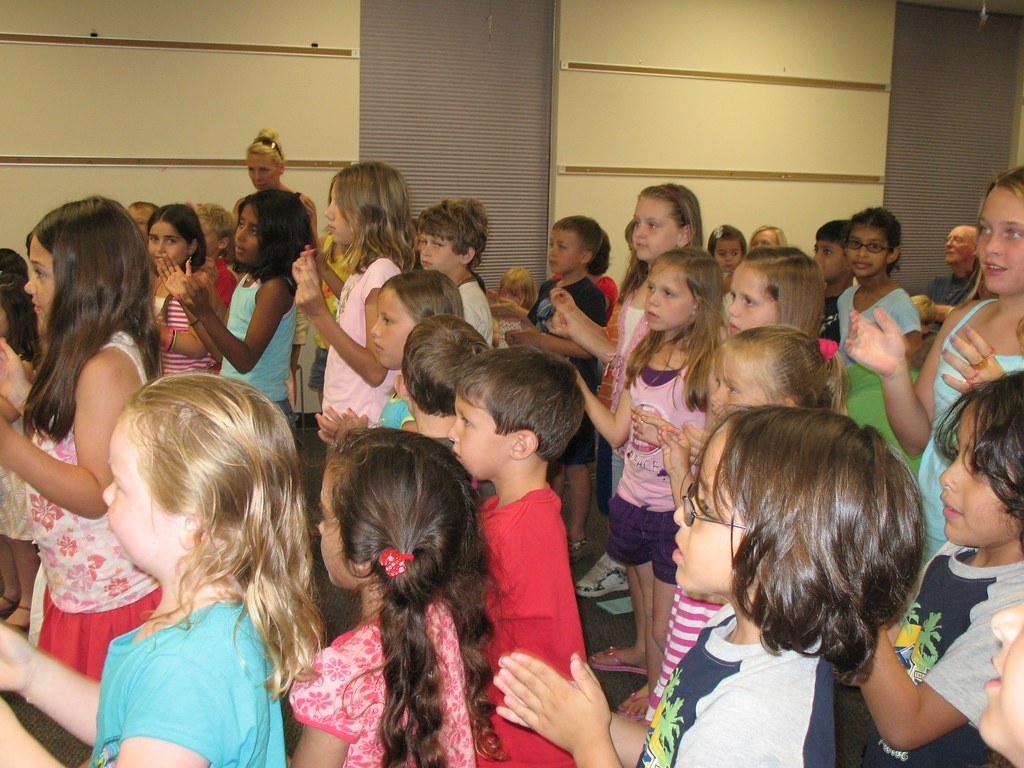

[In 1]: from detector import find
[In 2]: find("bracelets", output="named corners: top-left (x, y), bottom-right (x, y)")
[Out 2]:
top-left (190, 319), bottom-right (199, 326)
top-left (165, 328), bottom-right (177, 352)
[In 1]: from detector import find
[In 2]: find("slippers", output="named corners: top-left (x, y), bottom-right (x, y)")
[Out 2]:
top-left (616, 690), bottom-right (650, 721)
top-left (588, 645), bottom-right (648, 675)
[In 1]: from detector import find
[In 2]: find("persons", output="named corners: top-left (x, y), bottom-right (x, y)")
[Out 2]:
top-left (0, 129), bottom-right (1024, 768)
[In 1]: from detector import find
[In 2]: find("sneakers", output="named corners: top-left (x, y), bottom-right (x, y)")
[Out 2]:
top-left (575, 556), bottom-right (630, 598)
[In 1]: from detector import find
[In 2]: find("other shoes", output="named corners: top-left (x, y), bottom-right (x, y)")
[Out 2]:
top-left (4, 606), bottom-right (31, 634)
top-left (0, 594), bottom-right (21, 616)
top-left (568, 535), bottom-right (592, 564)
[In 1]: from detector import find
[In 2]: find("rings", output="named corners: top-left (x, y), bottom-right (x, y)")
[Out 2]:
top-left (641, 421), bottom-right (643, 424)
top-left (971, 356), bottom-right (988, 371)
top-left (986, 347), bottom-right (995, 360)
top-left (967, 370), bottom-right (979, 384)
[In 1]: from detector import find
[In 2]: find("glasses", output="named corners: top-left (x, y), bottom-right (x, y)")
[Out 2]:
top-left (252, 136), bottom-right (283, 160)
top-left (843, 239), bottom-right (892, 253)
top-left (682, 482), bottom-right (747, 531)
